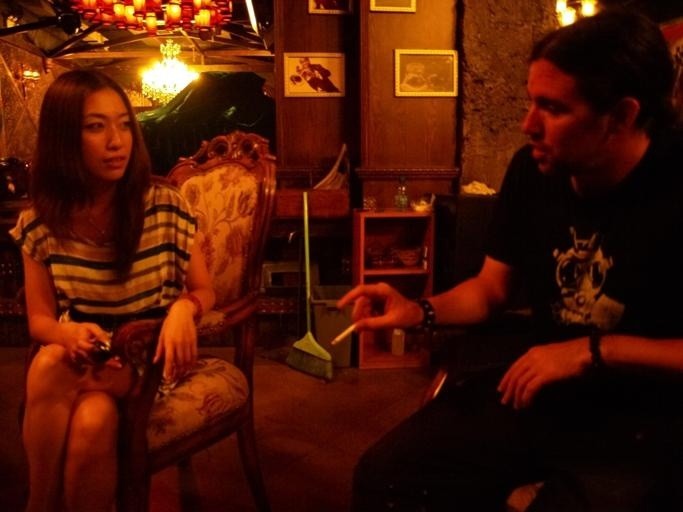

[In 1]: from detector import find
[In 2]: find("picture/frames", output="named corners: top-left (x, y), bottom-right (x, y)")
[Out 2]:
top-left (283, 0), bottom-right (460, 98)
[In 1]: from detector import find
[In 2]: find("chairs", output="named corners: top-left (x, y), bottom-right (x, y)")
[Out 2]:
top-left (18, 132), bottom-right (276, 512)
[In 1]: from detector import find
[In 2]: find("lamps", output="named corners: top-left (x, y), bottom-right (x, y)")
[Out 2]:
top-left (75, 0), bottom-right (226, 107)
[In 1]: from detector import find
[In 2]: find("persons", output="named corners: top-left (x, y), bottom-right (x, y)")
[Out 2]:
top-left (294, 57), bottom-right (339, 93)
top-left (329, 10), bottom-right (683, 511)
top-left (7, 65), bottom-right (217, 510)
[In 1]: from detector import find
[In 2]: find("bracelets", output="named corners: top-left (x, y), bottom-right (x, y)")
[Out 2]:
top-left (411, 297), bottom-right (435, 337)
top-left (587, 326), bottom-right (620, 370)
top-left (165, 292), bottom-right (204, 328)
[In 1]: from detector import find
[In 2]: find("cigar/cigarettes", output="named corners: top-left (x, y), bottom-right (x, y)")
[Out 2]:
top-left (330, 322), bottom-right (355, 346)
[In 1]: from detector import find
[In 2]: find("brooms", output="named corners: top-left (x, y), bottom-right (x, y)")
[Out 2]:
top-left (287, 191), bottom-right (333, 382)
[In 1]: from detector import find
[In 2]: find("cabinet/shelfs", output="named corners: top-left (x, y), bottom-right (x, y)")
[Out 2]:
top-left (352, 208), bottom-right (435, 369)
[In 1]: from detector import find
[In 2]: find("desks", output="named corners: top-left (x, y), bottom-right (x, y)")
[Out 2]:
top-left (277, 185), bottom-right (351, 220)
